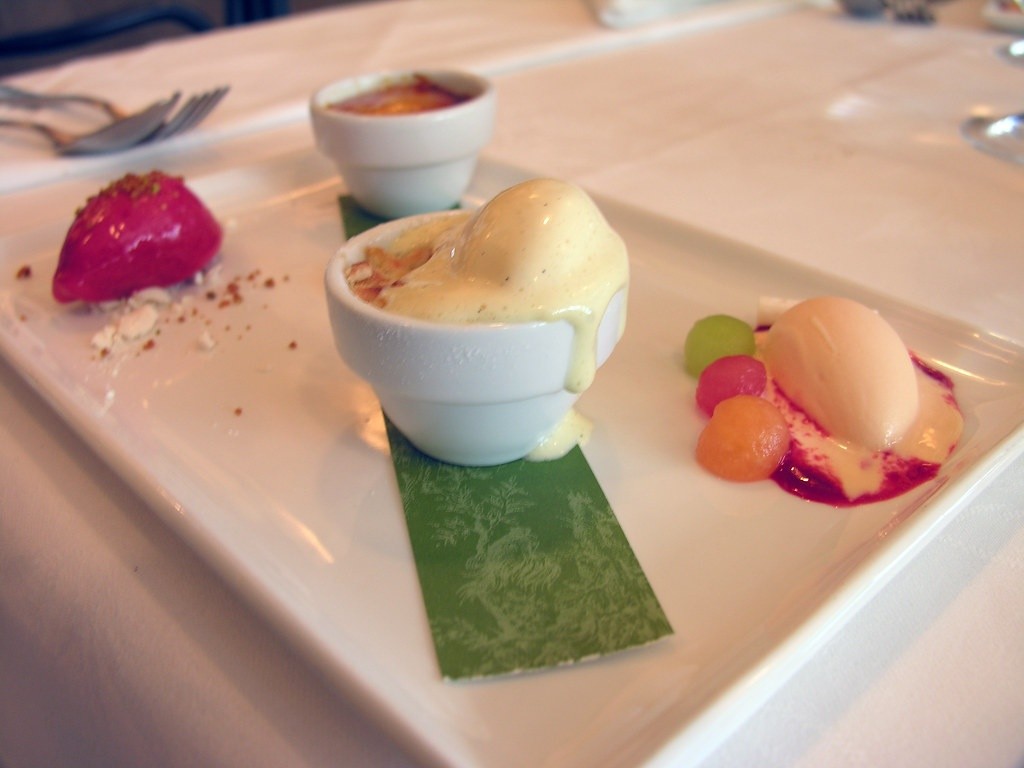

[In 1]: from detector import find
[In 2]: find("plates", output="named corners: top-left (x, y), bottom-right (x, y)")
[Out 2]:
top-left (2, 132), bottom-right (1021, 768)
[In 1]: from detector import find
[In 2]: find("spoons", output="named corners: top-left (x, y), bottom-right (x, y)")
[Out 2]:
top-left (1, 90), bottom-right (181, 159)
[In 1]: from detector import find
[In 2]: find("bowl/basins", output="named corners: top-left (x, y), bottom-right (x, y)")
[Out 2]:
top-left (310, 62), bottom-right (499, 220)
top-left (325, 209), bottom-right (629, 466)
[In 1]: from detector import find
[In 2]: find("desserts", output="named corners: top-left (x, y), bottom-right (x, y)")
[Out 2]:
top-left (683, 296), bottom-right (969, 512)
top-left (44, 169), bottom-right (224, 349)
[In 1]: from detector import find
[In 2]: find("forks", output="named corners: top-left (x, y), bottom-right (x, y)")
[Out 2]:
top-left (2, 73), bottom-right (228, 144)
